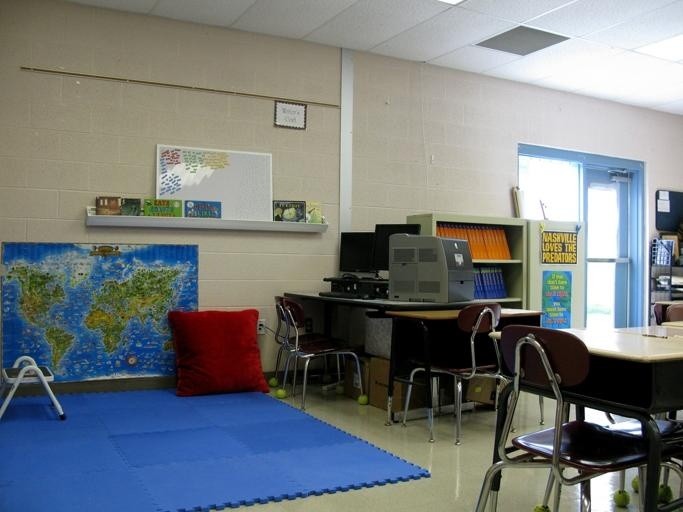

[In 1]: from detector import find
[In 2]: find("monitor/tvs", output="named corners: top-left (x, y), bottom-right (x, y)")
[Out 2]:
top-left (340, 232), bottom-right (378, 274)
top-left (374, 223), bottom-right (421, 270)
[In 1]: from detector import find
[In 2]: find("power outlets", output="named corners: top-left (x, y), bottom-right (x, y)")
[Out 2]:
top-left (258, 319), bottom-right (264, 334)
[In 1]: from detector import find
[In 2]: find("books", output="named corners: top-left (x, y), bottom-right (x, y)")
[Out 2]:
top-left (473, 266), bottom-right (507, 300)
top-left (436, 222), bottom-right (512, 259)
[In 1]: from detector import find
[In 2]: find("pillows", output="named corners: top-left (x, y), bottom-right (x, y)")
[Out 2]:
top-left (166, 308), bottom-right (270, 395)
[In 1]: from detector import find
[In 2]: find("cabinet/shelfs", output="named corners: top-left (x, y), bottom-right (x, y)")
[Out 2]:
top-left (405, 211), bottom-right (528, 312)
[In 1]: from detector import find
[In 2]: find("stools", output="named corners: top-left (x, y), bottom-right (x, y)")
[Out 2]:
top-left (0, 356), bottom-right (63, 421)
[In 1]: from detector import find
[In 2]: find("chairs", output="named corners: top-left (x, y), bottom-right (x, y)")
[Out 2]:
top-left (407, 305), bottom-right (517, 445)
top-left (650, 304), bottom-right (683, 322)
top-left (473, 320), bottom-right (681, 512)
top-left (272, 296), bottom-right (369, 414)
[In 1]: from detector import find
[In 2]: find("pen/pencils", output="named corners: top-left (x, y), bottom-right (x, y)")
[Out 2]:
top-left (642, 334), bottom-right (668, 339)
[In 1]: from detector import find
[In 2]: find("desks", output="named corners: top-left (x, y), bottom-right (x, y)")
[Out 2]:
top-left (288, 291), bottom-right (537, 427)
top-left (530, 320), bottom-right (683, 442)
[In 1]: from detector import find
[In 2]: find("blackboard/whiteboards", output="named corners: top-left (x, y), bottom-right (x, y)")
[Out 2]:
top-left (155, 144), bottom-right (273, 222)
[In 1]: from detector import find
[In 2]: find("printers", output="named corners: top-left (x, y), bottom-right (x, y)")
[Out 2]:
top-left (388, 233), bottom-right (475, 303)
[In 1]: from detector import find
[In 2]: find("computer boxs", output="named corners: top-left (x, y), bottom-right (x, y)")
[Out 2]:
top-left (330, 276), bottom-right (358, 295)
top-left (358, 278), bottom-right (389, 299)
